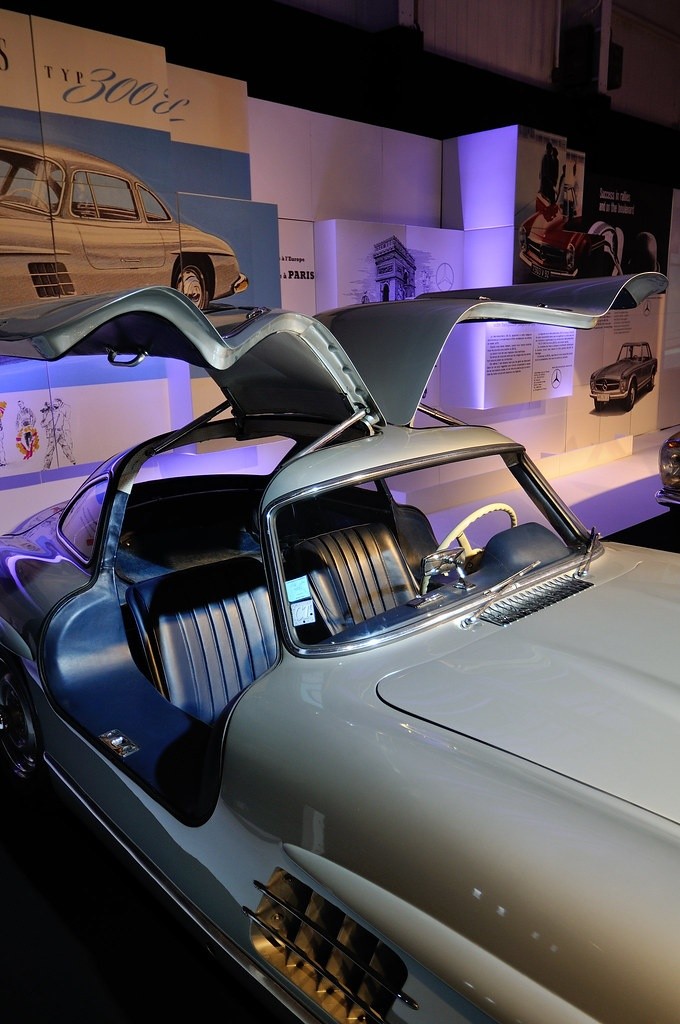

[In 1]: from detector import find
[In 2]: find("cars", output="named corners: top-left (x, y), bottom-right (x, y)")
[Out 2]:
top-left (517, 183), bottom-right (616, 284)
top-left (589, 341), bottom-right (657, 412)
top-left (654, 430), bottom-right (680, 516)
top-left (0, 137), bottom-right (251, 317)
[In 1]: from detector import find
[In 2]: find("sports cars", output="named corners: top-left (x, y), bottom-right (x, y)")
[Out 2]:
top-left (0, 270), bottom-right (680, 1024)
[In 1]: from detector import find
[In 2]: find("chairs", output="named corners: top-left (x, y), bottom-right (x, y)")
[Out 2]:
top-left (125, 554), bottom-right (277, 727)
top-left (295, 519), bottom-right (425, 637)
